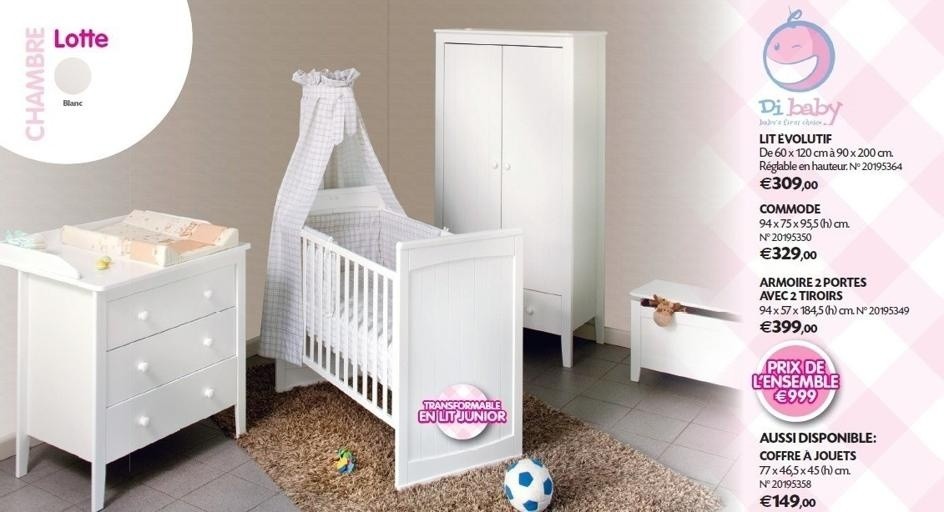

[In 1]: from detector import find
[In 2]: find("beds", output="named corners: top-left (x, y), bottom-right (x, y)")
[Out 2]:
top-left (276, 186), bottom-right (526, 490)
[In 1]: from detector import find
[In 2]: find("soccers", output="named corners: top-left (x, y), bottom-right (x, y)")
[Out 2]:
top-left (502, 455), bottom-right (555, 512)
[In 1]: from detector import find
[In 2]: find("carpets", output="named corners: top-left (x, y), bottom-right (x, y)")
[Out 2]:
top-left (213, 371), bottom-right (727, 512)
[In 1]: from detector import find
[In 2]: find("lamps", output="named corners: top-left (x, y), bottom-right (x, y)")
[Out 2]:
top-left (1, 0), bottom-right (193, 164)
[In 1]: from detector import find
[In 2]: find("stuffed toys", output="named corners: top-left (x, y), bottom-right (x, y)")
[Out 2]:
top-left (642, 292), bottom-right (690, 324)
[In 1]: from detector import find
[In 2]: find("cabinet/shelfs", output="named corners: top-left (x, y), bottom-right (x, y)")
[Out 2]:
top-left (433, 29), bottom-right (608, 367)
top-left (1, 210), bottom-right (249, 512)
top-left (631, 281), bottom-right (748, 392)
